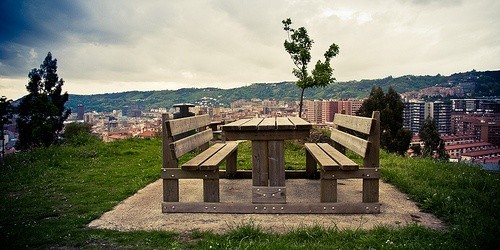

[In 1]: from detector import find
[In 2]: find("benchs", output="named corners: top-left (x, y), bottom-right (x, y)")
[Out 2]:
top-left (160, 112), bottom-right (240, 214)
top-left (302, 111), bottom-right (383, 215)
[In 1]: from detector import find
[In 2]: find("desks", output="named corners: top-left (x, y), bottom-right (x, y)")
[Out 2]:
top-left (221, 117), bottom-right (313, 214)
